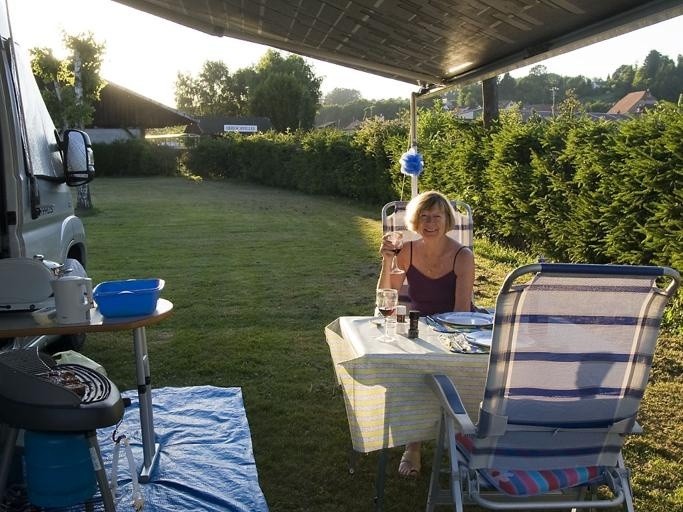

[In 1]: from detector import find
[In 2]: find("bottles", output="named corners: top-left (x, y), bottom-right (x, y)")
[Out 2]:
top-left (394, 304), bottom-right (419, 339)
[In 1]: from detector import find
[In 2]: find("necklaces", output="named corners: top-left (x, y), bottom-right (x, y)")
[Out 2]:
top-left (424, 267), bottom-right (433, 275)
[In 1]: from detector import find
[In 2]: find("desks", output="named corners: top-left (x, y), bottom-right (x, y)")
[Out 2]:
top-left (0, 296), bottom-right (174, 483)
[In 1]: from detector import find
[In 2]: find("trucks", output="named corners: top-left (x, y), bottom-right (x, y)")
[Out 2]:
top-left (0, 0), bottom-right (97, 512)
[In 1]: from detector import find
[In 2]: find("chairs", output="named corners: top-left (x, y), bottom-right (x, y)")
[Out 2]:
top-left (423, 262), bottom-right (682, 510)
top-left (381, 199), bottom-right (474, 313)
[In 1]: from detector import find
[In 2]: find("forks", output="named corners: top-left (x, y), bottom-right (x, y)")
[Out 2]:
top-left (447, 335), bottom-right (466, 353)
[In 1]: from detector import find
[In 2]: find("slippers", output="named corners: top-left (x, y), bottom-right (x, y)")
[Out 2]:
top-left (400, 446), bottom-right (422, 476)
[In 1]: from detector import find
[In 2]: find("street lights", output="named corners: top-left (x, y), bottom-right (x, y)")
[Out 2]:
top-left (549, 86), bottom-right (560, 118)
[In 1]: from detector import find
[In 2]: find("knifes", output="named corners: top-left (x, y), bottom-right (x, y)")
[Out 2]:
top-left (426, 315), bottom-right (447, 331)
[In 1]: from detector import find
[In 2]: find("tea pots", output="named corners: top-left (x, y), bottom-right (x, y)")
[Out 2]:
top-left (47, 275), bottom-right (95, 325)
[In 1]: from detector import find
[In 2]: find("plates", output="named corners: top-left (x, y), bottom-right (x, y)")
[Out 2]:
top-left (369, 317), bottom-right (385, 325)
top-left (437, 311), bottom-right (495, 326)
top-left (465, 330), bottom-right (493, 348)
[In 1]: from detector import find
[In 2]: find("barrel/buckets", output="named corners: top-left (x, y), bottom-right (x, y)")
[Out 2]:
top-left (23, 430), bottom-right (97, 508)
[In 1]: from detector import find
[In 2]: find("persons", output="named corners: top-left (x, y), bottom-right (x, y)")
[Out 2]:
top-left (374, 188), bottom-right (490, 481)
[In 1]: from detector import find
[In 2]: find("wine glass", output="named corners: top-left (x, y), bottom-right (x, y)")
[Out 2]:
top-left (385, 232), bottom-right (406, 275)
top-left (376, 288), bottom-right (398, 344)
